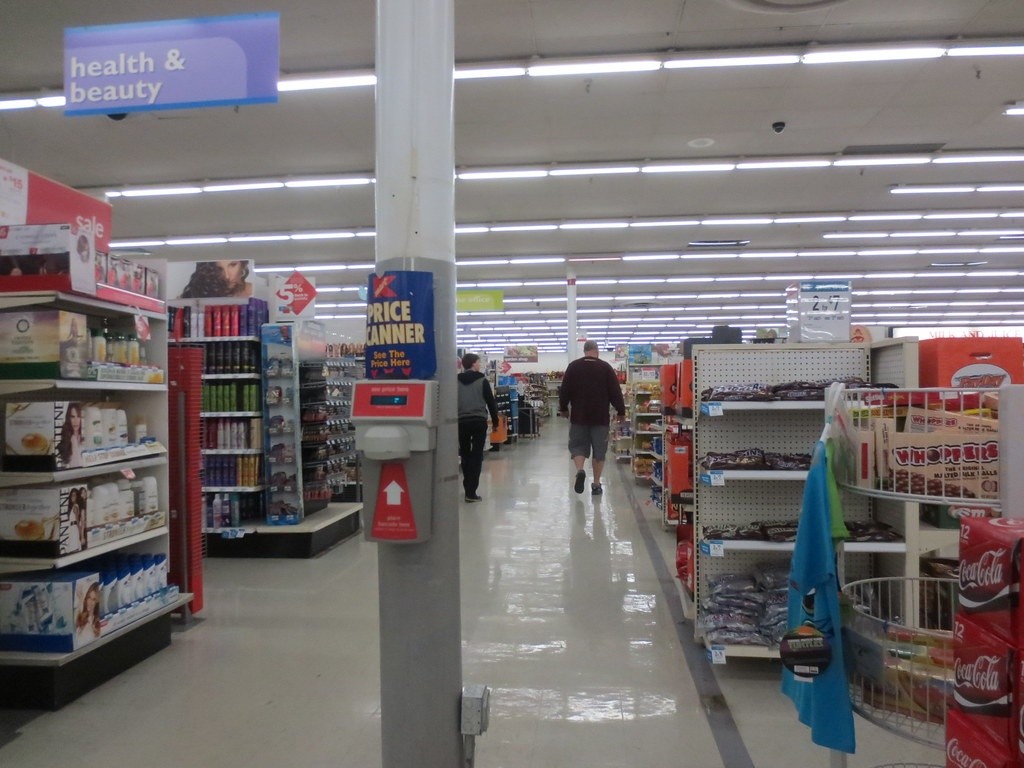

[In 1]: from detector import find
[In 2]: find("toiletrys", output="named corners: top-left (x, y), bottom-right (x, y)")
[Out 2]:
top-left (94, 552), bottom-right (168, 617)
top-left (77, 475), bottom-right (159, 528)
top-left (84, 315), bottom-right (151, 367)
top-left (169, 298), bottom-right (267, 528)
top-left (76, 397), bottom-right (149, 452)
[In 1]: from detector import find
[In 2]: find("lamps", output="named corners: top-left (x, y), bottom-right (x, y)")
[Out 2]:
top-left (0, 35), bottom-right (1024, 353)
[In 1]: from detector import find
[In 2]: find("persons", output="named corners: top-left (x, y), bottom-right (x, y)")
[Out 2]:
top-left (181, 260), bottom-right (253, 298)
top-left (55, 403), bottom-right (83, 468)
top-left (75, 582), bottom-right (101, 650)
top-left (558, 340), bottom-right (625, 494)
top-left (65, 487), bottom-right (87, 556)
top-left (457, 353), bottom-right (500, 502)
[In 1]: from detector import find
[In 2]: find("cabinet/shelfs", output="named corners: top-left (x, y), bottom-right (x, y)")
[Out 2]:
top-left (168, 297), bottom-right (518, 559)
top-left (610, 338), bottom-right (919, 659)
top-left (0, 222), bottom-right (196, 712)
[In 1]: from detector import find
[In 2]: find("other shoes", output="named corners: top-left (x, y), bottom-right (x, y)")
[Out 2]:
top-left (466, 491), bottom-right (483, 502)
top-left (574, 470), bottom-right (586, 494)
top-left (591, 483), bottom-right (602, 495)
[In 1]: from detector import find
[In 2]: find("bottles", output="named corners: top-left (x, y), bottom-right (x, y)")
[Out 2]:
top-left (86, 332), bottom-right (148, 367)
top-left (213, 494), bottom-right (240, 527)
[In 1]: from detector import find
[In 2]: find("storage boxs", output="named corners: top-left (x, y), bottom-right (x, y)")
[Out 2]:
top-left (0, 484), bottom-right (88, 559)
top-left (686, 541), bottom-right (694, 602)
top-left (4, 401), bottom-right (83, 472)
top-left (947, 518), bottom-right (1024, 768)
top-left (675, 359), bottom-right (692, 418)
top-left (844, 393), bottom-right (1000, 501)
top-left (1, 571), bottom-right (101, 652)
top-left (660, 365), bottom-right (675, 416)
top-left (666, 433), bottom-right (693, 505)
top-left (919, 337), bottom-right (1024, 411)
top-left (1, 310), bottom-right (89, 381)
top-left (1, 224), bottom-right (98, 299)
top-left (922, 503), bottom-right (1002, 529)
top-left (919, 562), bottom-right (956, 632)
top-left (168, 345), bottom-right (206, 614)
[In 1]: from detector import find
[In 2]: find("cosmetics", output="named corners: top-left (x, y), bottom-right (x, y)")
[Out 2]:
top-left (166, 297), bottom-right (272, 528)
top-left (84, 327), bottom-right (149, 367)
